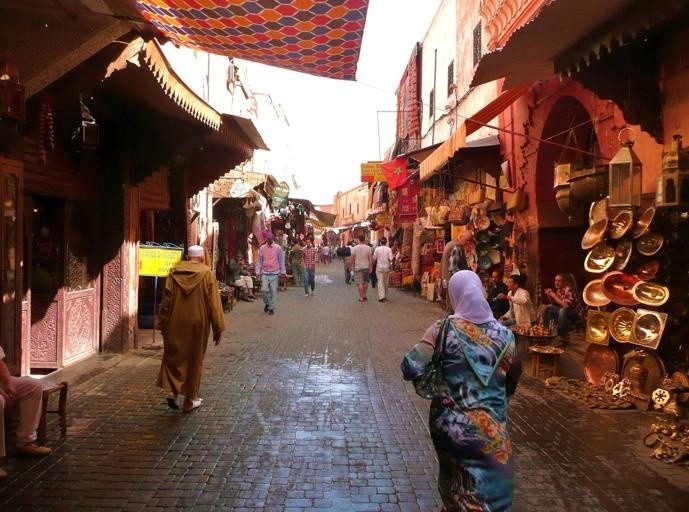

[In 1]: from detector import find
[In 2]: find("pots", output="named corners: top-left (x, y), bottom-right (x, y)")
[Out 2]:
top-left (566, 171), bottom-right (609, 204)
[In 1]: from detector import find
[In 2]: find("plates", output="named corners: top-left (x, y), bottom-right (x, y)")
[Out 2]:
top-left (474, 213), bottom-right (506, 271)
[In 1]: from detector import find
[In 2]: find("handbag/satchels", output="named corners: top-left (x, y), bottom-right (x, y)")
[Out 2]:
top-left (413, 351), bottom-right (442, 399)
top-left (449, 210), bottom-right (467, 222)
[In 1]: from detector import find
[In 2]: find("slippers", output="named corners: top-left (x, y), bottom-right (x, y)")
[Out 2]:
top-left (183, 401), bottom-right (201, 413)
top-left (166, 397), bottom-right (179, 410)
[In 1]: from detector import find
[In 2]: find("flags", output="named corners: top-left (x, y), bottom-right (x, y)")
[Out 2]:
top-left (380, 158), bottom-right (409, 190)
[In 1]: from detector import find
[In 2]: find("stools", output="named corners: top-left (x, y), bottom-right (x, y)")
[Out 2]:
top-left (6, 381), bottom-right (69, 446)
top-left (528, 344), bottom-right (565, 378)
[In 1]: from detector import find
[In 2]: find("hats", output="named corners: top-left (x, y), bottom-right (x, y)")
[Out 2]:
top-left (188, 245), bottom-right (204, 258)
top-left (266, 232), bottom-right (274, 238)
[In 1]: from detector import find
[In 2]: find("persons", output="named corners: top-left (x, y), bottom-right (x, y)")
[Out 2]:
top-left (0, 343), bottom-right (53, 478)
top-left (438, 230), bottom-right (474, 317)
top-left (228, 248), bottom-right (257, 302)
top-left (542, 273), bottom-right (587, 347)
top-left (154, 244), bottom-right (227, 414)
top-left (495, 271), bottom-right (538, 345)
top-left (400, 269), bottom-right (525, 512)
top-left (485, 269), bottom-right (510, 321)
top-left (288, 232), bottom-right (396, 303)
top-left (254, 232), bottom-right (287, 316)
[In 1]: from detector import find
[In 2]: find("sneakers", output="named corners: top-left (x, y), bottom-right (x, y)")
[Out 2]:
top-left (242, 297), bottom-right (250, 302)
top-left (359, 298), bottom-right (363, 302)
top-left (383, 298), bottom-right (387, 300)
top-left (363, 296), bottom-right (367, 300)
top-left (311, 288), bottom-right (314, 291)
top-left (264, 304), bottom-right (269, 312)
top-left (248, 295), bottom-right (256, 299)
top-left (346, 282), bottom-right (351, 285)
top-left (305, 292), bottom-right (309, 297)
top-left (378, 299), bottom-right (383, 302)
top-left (269, 309), bottom-right (274, 315)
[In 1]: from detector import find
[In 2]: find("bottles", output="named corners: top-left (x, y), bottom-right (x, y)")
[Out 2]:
top-left (350, 270), bottom-right (355, 278)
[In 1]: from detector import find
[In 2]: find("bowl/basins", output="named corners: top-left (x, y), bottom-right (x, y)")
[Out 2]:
top-left (580, 208), bottom-right (670, 351)
top-left (529, 346), bottom-right (565, 357)
